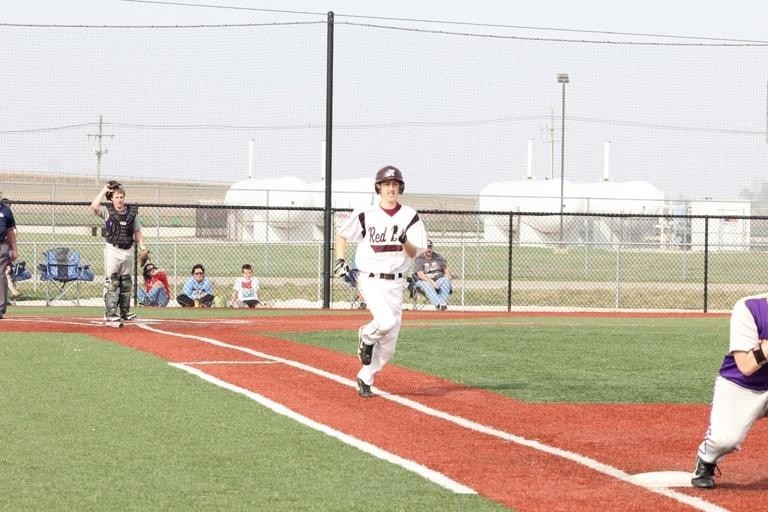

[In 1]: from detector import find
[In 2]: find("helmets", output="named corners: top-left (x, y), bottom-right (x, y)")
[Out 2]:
top-left (106, 181), bottom-right (121, 200)
top-left (375, 166), bottom-right (405, 195)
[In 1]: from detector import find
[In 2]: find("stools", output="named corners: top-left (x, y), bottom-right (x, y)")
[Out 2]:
top-left (410, 278), bottom-right (451, 310)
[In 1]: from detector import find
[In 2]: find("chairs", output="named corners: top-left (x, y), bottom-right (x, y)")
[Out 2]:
top-left (10, 255), bottom-right (32, 302)
top-left (341, 267), bottom-right (366, 312)
top-left (38, 246), bottom-right (96, 306)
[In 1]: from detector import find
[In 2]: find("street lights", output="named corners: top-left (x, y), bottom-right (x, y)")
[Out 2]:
top-left (554, 72), bottom-right (571, 246)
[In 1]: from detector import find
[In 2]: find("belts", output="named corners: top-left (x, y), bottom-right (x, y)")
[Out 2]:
top-left (369, 273), bottom-right (402, 279)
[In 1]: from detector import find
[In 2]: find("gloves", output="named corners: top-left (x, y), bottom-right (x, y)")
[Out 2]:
top-left (391, 226), bottom-right (407, 243)
top-left (334, 259), bottom-right (349, 278)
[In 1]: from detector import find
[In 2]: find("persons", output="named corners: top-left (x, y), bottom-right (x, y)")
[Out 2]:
top-left (413, 240), bottom-right (452, 311)
top-left (136, 263), bottom-right (170, 308)
top-left (90, 182), bottom-right (151, 322)
top-left (0, 192), bottom-right (18, 319)
top-left (691, 294), bottom-right (768, 489)
top-left (346, 255), bottom-right (367, 309)
top-left (1, 198), bottom-right (21, 307)
top-left (334, 166), bottom-right (428, 397)
top-left (230, 264), bottom-right (263, 309)
top-left (176, 264), bottom-right (215, 309)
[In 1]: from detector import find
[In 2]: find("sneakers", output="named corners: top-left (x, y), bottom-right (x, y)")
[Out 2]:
top-left (692, 457), bottom-right (722, 488)
top-left (356, 377), bottom-right (374, 397)
top-left (104, 314), bottom-right (121, 321)
top-left (359, 326), bottom-right (374, 364)
top-left (121, 314), bottom-right (135, 320)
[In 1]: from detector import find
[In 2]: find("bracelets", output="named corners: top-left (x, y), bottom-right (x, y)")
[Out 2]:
top-left (752, 344), bottom-right (767, 366)
